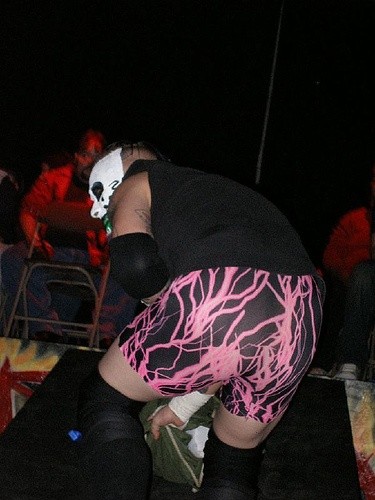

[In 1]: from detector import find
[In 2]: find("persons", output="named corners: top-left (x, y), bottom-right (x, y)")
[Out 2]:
top-left (259, 180), bottom-right (332, 379)
top-left (325, 177), bottom-right (375, 381)
top-left (1, 123), bottom-right (138, 350)
top-left (77, 141), bottom-right (323, 500)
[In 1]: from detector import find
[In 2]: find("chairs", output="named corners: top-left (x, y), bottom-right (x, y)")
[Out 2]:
top-left (3, 204), bottom-right (112, 349)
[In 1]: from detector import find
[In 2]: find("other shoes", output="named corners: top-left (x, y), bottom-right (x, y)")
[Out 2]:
top-left (307, 365), bottom-right (330, 380)
top-left (334, 363), bottom-right (361, 381)
top-left (88, 324), bottom-right (117, 348)
top-left (34, 317), bottom-right (66, 344)
